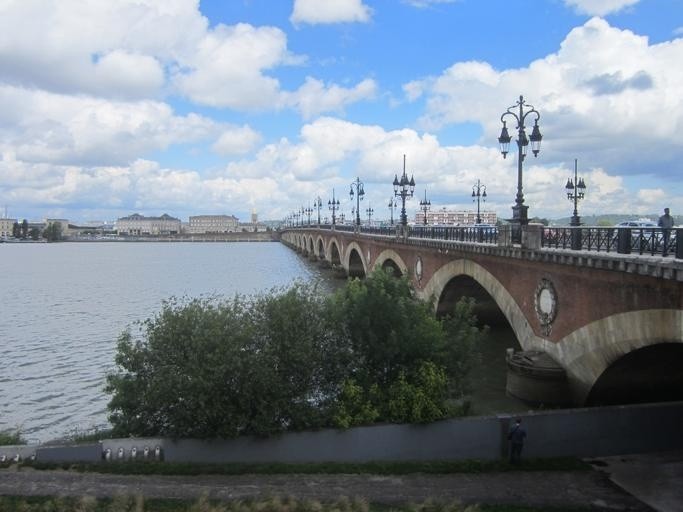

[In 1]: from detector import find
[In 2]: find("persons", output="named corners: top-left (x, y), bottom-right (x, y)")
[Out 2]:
top-left (504, 415), bottom-right (527, 467)
top-left (658, 207), bottom-right (675, 254)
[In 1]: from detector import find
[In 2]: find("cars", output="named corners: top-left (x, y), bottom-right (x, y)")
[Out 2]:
top-left (378, 222), bottom-right (561, 244)
top-left (611, 221), bottom-right (676, 251)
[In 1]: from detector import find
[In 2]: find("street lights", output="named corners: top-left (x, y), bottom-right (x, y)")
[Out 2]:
top-left (275, 198), bottom-right (345, 228)
top-left (347, 180), bottom-right (364, 225)
top-left (495, 95), bottom-right (543, 228)
top-left (392, 173), bottom-right (415, 235)
top-left (565, 177), bottom-right (587, 247)
top-left (470, 184), bottom-right (487, 221)
top-left (365, 205), bottom-right (375, 223)
top-left (419, 199), bottom-right (431, 223)
